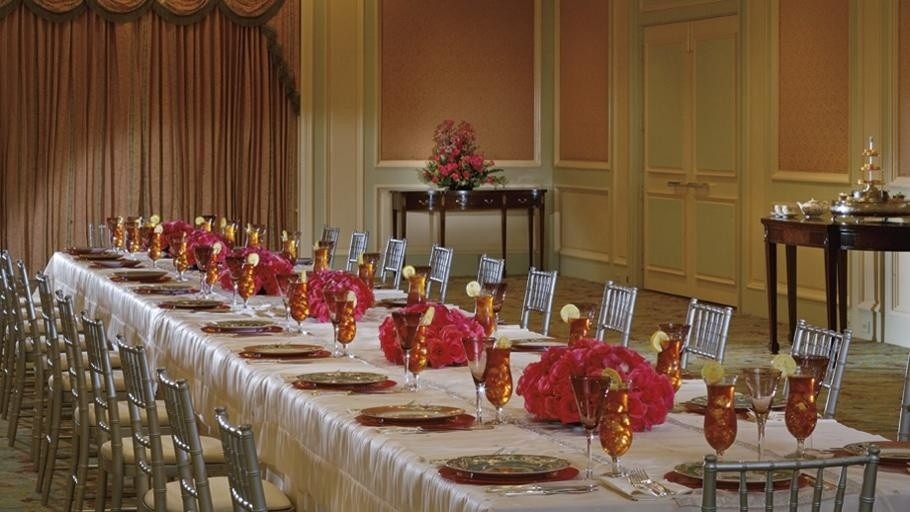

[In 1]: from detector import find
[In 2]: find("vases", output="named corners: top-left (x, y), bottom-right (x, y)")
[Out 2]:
top-left (449, 178), bottom-right (472, 192)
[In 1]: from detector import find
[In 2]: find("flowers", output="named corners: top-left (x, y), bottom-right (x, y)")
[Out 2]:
top-left (411, 118), bottom-right (511, 195)
top-left (160, 221), bottom-right (292, 298)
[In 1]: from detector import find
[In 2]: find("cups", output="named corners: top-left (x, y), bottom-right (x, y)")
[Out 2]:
top-left (563, 301), bottom-right (597, 346)
top-left (770, 202), bottom-right (794, 219)
top-left (654, 321), bottom-right (691, 395)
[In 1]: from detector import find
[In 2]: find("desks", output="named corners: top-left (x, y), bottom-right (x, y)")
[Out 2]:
top-left (389, 188), bottom-right (551, 283)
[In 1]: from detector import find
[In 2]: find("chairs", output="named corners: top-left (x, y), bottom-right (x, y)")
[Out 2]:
top-left (697, 443), bottom-right (882, 512)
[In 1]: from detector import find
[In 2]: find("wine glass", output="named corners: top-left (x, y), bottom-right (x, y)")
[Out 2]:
top-left (565, 367), bottom-right (633, 478)
top-left (460, 334), bottom-right (515, 430)
top-left (785, 352), bottom-right (831, 462)
top-left (107, 202), bottom-right (508, 397)
top-left (702, 365), bottom-right (782, 476)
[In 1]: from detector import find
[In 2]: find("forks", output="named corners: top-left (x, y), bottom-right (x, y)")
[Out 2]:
top-left (622, 465), bottom-right (676, 497)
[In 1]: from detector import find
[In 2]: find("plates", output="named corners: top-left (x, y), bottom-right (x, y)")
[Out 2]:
top-left (841, 438), bottom-right (910, 465)
top-left (359, 403), bottom-right (468, 424)
top-left (672, 458), bottom-right (794, 485)
top-left (442, 451), bottom-right (572, 477)
top-left (684, 392), bottom-right (788, 414)
top-left (61, 242), bottom-right (388, 387)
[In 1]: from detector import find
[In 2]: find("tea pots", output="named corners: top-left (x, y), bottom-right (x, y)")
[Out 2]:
top-left (796, 197), bottom-right (830, 218)
